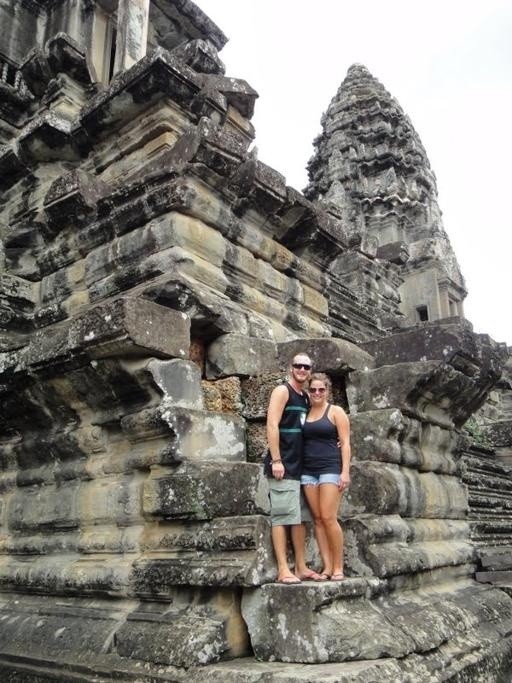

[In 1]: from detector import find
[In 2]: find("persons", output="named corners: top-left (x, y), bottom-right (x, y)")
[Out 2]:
top-left (263, 352), bottom-right (342, 584)
top-left (300, 371), bottom-right (350, 581)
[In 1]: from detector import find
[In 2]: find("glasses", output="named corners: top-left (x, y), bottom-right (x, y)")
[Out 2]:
top-left (308, 386), bottom-right (326, 393)
top-left (291, 363), bottom-right (313, 371)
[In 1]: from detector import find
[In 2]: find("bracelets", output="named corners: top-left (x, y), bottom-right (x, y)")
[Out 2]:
top-left (269, 459), bottom-right (282, 463)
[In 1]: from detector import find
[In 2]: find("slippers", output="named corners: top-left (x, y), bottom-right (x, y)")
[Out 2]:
top-left (275, 575), bottom-right (302, 584)
top-left (296, 572), bottom-right (329, 582)
top-left (330, 573), bottom-right (346, 581)
top-left (317, 572), bottom-right (331, 582)
